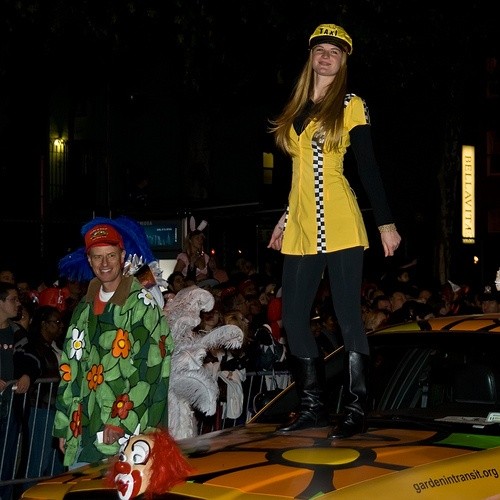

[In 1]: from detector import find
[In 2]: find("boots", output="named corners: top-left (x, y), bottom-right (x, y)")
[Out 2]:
top-left (274, 355), bottom-right (332, 435)
top-left (327, 349), bottom-right (372, 441)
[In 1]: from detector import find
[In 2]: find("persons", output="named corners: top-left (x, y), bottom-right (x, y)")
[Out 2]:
top-left (266, 24), bottom-right (401, 439)
top-left (52, 224), bottom-right (175, 471)
top-left (0, 230), bottom-right (500, 500)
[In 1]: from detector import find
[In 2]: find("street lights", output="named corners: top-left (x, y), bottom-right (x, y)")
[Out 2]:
top-left (39, 133), bottom-right (67, 275)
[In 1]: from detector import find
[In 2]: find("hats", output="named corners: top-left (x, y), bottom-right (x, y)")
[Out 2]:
top-left (309, 25), bottom-right (353, 55)
top-left (85, 224), bottom-right (124, 251)
top-left (240, 279), bottom-right (255, 288)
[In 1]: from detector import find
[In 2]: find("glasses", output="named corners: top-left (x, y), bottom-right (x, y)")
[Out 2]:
top-left (18, 287), bottom-right (29, 293)
top-left (3, 296), bottom-right (20, 303)
top-left (45, 319), bottom-right (64, 325)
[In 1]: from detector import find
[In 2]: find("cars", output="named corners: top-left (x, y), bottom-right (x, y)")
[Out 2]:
top-left (19, 314), bottom-right (500, 500)
